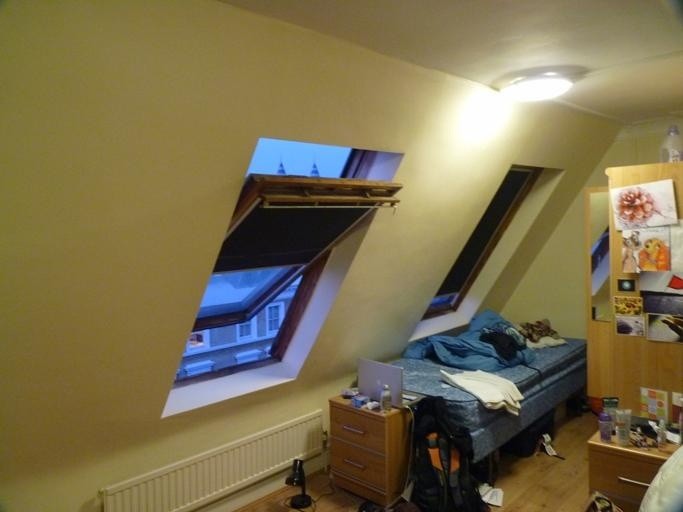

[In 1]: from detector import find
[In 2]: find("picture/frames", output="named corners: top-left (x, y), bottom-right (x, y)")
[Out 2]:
top-left (645, 313), bottom-right (683, 344)
top-left (614, 314), bottom-right (645, 337)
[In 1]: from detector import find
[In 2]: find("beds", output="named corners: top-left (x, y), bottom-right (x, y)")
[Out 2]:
top-left (387, 336), bottom-right (586, 487)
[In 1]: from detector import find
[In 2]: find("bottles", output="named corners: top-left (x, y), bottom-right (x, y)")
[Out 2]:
top-left (596, 412), bottom-right (613, 445)
top-left (380, 384), bottom-right (392, 414)
top-left (615, 421), bottom-right (630, 449)
top-left (655, 419), bottom-right (667, 450)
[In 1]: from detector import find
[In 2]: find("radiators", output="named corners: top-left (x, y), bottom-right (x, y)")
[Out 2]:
top-left (99, 409), bottom-right (327, 512)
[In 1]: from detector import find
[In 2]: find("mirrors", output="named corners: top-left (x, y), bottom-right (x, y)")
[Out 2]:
top-left (589, 192), bottom-right (610, 323)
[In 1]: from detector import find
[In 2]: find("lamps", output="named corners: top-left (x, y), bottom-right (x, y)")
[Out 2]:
top-left (500, 72), bottom-right (573, 103)
top-left (285, 460), bottom-right (312, 509)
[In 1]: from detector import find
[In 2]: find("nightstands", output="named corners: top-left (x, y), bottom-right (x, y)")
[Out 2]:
top-left (328, 393), bottom-right (426, 507)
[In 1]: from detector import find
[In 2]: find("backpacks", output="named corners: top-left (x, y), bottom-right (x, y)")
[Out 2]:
top-left (409, 406), bottom-right (489, 512)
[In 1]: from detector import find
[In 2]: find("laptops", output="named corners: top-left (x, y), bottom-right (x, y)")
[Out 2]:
top-left (357, 356), bottom-right (427, 409)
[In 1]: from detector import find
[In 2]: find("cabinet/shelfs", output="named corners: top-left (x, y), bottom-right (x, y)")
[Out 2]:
top-left (586, 161), bottom-right (683, 423)
top-left (586, 428), bottom-right (683, 512)
